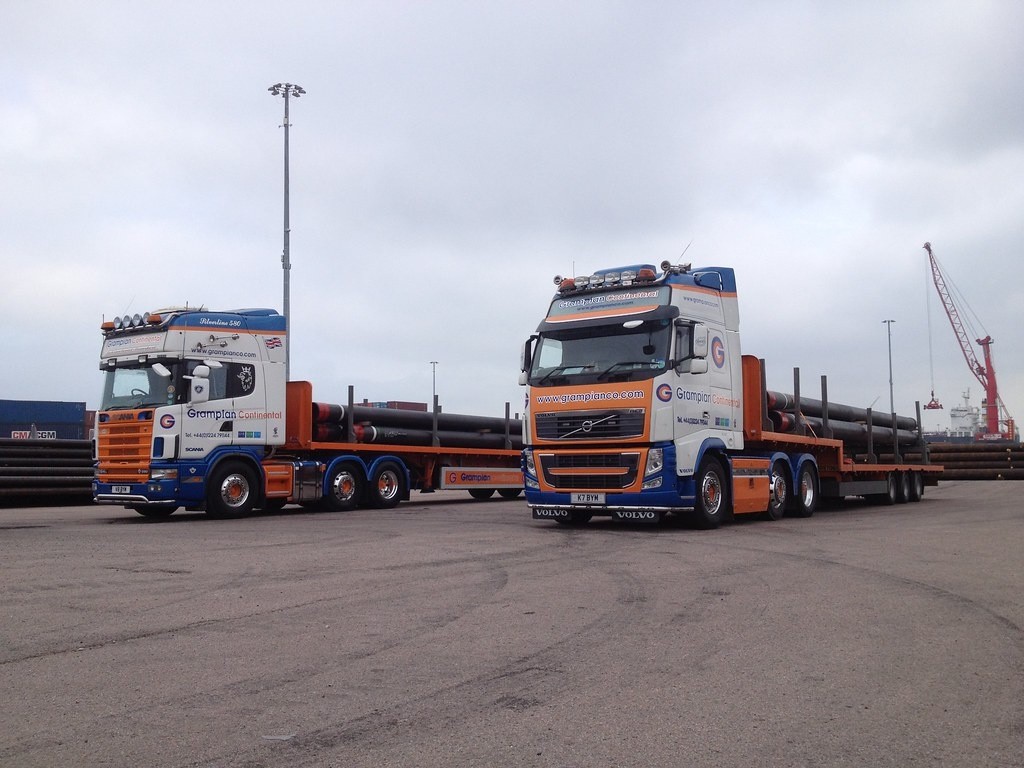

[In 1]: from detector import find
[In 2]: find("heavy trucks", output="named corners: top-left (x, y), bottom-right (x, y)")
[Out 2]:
top-left (518, 261), bottom-right (944, 530)
top-left (92, 307), bottom-right (523, 518)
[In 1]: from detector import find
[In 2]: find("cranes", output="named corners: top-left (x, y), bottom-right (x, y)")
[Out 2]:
top-left (923, 242), bottom-right (1015, 442)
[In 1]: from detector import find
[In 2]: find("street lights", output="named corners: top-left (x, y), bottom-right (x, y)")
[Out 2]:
top-left (430, 361), bottom-right (438, 395)
top-left (882, 319), bottom-right (895, 413)
top-left (268, 83), bottom-right (306, 383)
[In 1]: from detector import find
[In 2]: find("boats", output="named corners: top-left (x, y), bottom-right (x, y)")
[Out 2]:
top-left (922, 387), bottom-right (980, 442)
